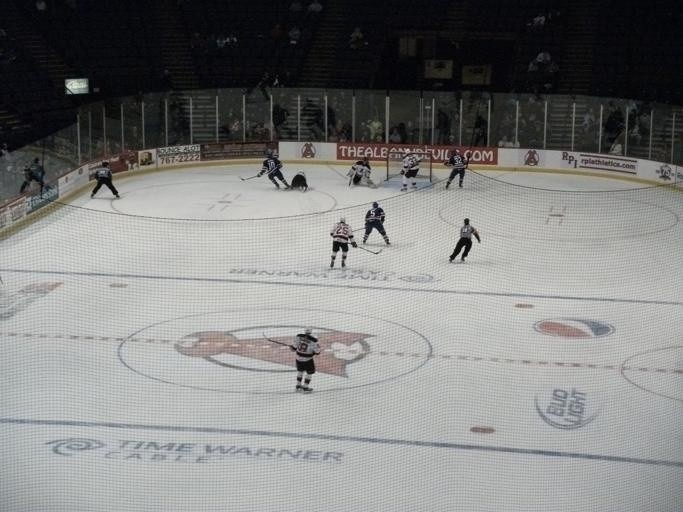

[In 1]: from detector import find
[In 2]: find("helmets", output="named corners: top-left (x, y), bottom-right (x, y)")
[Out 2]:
top-left (304, 326), bottom-right (312, 335)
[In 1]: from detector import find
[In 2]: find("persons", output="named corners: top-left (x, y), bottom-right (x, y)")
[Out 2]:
top-left (445, 152), bottom-right (468, 187)
top-left (349, 158), bottom-right (371, 184)
top-left (19, 158), bottom-right (50, 193)
top-left (401, 150), bottom-right (419, 190)
top-left (291, 171), bottom-right (307, 188)
top-left (1, 1), bottom-right (651, 156)
top-left (289, 327), bottom-right (322, 391)
top-left (257, 153), bottom-right (289, 188)
top-left (363, 202), bottom-right (391, 244)
top-left (450, 219), bottom-right (481, 261)
top-left (331, 217), bottom-right (357, 267)
top-left (91, 162), bottom-right (120, 198)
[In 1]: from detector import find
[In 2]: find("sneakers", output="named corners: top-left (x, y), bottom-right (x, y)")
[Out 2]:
top-left (296, 384), bottom-right (301, 388)
top-left (304, 388), bottom-right (313, 391)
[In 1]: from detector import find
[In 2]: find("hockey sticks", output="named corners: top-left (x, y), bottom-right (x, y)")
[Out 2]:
top-left (349, 242), bottom-right (382, 255)
top-left (261, 333), bottom-right (290, 347)
top-left (238, 175), bottom-right (257, 181)
